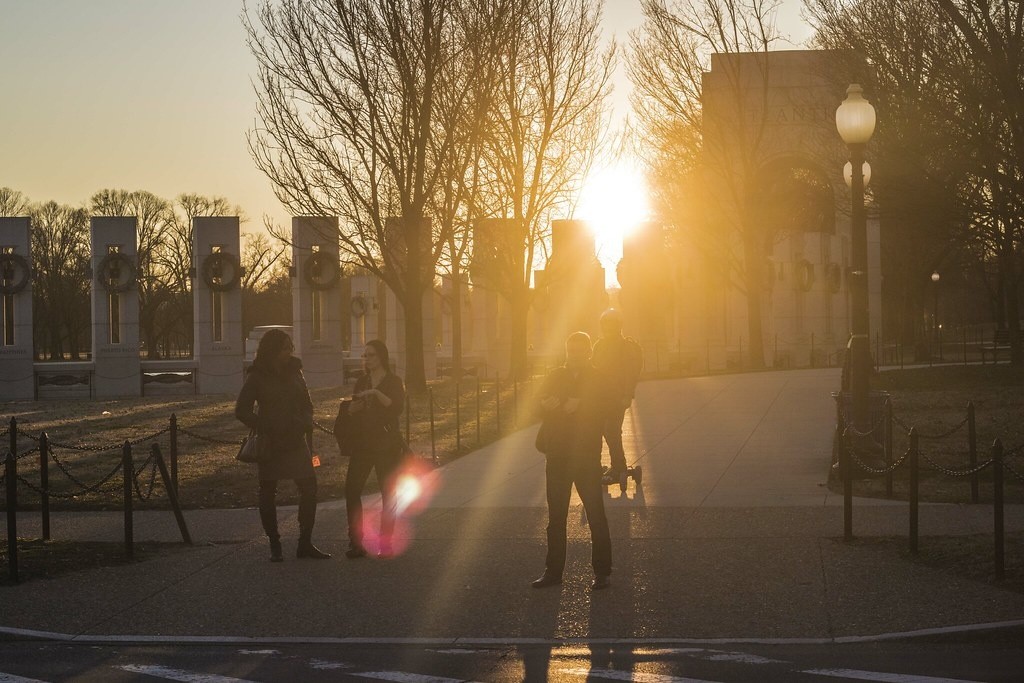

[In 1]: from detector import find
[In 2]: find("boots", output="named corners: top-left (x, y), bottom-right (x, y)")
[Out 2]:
top-left (260, 502), bottom-right (331, 562)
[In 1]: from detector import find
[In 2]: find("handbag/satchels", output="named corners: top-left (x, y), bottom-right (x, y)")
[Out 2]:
top-left (237, 428), bottom-right (266, 463)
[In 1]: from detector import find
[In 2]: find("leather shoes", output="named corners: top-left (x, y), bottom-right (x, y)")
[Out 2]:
top-left (532, 574), bottom-right (612, 590)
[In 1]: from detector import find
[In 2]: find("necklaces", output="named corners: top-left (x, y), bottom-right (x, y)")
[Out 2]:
top-left (362, 369), bottom-right (387, 411)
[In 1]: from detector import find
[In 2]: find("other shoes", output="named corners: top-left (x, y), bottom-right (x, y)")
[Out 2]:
top-left (346, 547), bottom-right (393, 559)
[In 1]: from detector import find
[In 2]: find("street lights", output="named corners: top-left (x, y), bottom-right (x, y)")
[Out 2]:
top-left (931, 271), bottom-right (941, 359)
top-left (840, 158), bottom-right (876, 394)
top-left (838, 83), bottom-right (890, 473)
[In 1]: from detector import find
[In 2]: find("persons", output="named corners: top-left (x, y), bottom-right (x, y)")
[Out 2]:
top-left (233, 328), bottom-right (331, 563)
top-left (585, 308), bottom-right (642, 496)
top-left (338, 339), bottom-right (408, 562)
top-left (530, 332), bottom-right (614, 592)
